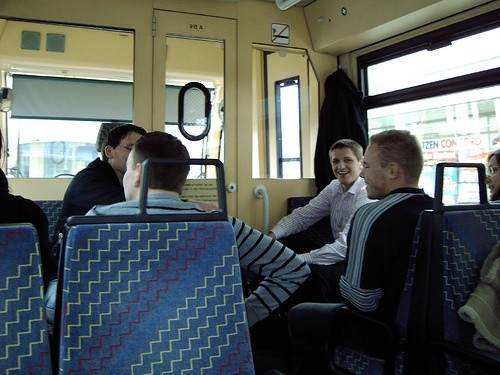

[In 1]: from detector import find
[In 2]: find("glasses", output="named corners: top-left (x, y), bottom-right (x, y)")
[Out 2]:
top-left (119, 144), bottom-right (133, 151)
top-left (486, 168), bottom-right (500, 175)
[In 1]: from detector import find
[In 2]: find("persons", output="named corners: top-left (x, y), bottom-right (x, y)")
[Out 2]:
top-left (0, 169), bottom-right (49, 291)
top-left (266, 139), bottom-right (380, 314)
top-left (49, 124), bottom-right (147, 279)
top-left (45, 132), bottom-right (313, 329)
top-left (486, 148), bottom-right (500, 201)
top-left (286, 130), bottom-right (445, 375)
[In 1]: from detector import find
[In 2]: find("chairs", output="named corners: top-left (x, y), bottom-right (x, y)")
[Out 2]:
top-left (1, 160), bottom-right (500, 375)
